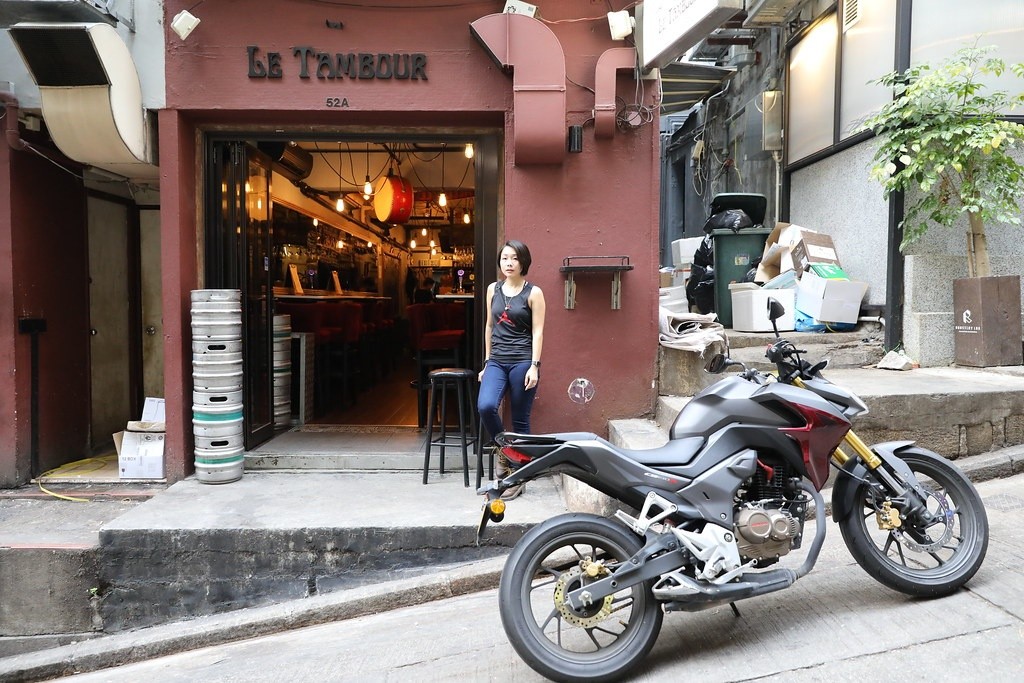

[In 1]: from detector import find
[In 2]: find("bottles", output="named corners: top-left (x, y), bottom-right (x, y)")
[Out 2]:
top-left (453, 247), bottom-right (474, 268)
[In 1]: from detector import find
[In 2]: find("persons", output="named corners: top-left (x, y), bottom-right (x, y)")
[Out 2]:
top-left (477, 240), bottom-right (546, 501)
top-left (414, 277), bottom-right (433, 304)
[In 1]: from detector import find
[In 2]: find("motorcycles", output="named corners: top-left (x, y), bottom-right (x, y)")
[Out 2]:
top-left (474, 295), bottom-right (990, 683)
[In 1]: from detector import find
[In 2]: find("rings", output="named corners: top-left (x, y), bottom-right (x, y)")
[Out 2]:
top-left (534, 384), bottom-right (536, 387)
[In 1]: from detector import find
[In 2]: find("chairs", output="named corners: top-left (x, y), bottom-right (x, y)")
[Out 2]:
top-left (276, 300), bottom-right (402, 416)
top-left (407, 302), bottom-right (468, 428)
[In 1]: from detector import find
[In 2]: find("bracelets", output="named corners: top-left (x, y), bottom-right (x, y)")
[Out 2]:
top-left (483, 360), bottom-right (489, 363)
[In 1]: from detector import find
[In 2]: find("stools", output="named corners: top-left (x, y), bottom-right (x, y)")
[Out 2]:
top-left (422, 368), bottom-right (485, 488)
top-left (475, 417), bottom-right (523, 497)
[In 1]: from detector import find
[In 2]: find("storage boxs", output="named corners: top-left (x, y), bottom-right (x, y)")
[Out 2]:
top-left (112, 397), bottom-right (166, 479)
top-left (659, 222), bottom-right (869, 332)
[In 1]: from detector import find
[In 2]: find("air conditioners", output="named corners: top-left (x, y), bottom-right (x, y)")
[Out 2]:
top-left (257, 141), bottom-right (313, 179)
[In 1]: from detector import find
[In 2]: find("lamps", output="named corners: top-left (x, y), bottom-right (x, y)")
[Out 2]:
top-left (169, 9), bottom-right (201, 41)
top-left (607, 11), bottom-right (636, 41)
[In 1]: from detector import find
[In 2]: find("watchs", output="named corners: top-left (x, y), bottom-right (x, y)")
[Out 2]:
top-left (532, 360), bottom-right (541, 367)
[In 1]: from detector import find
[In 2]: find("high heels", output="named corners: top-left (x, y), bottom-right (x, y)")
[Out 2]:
top-left (491, 446), bottom-right (510, 479)
top-left (500, 467), bottom-right (526, 499)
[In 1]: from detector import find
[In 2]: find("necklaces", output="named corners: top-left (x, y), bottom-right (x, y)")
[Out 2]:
top-left (504, 286), bottom-right (518, 310)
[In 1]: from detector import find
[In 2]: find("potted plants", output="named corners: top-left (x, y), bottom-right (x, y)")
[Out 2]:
top-left (843, 29), bottom-right (1023, 368)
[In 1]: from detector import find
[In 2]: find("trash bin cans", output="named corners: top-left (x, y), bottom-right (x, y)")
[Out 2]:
top-left (708, 193), bottom-right (773, 330)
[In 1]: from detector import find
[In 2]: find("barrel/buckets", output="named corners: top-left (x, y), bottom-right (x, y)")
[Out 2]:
top-left (191, 289), bottom-right (244, 484)
top-left (273, 315), bottom-right (292, 428)
